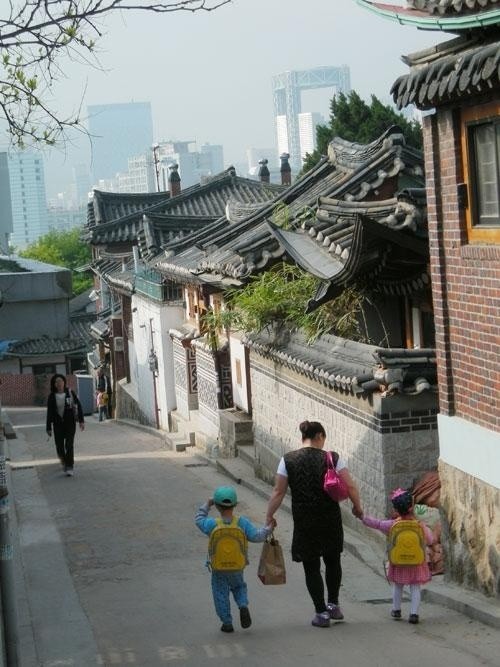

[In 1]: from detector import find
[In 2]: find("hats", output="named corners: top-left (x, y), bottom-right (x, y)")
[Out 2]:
top-left (212, 485), bottom-right (238, 509)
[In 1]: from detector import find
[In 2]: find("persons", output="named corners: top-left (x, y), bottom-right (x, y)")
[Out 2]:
top-left (97, 369), bottom-right (113, 419)
top-left (194, 486), bottom-right (278, 632)
top-left (266, 420), bottom-right (362, 626)
top-left (95, 384), bottom-right (110, 421)
top-left (360, 488), bottom-right (434, 623)
top-left (46, 373), bottom-right (85, 476)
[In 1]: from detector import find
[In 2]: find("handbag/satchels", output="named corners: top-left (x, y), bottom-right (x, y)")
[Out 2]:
top-left (256, 532), bottom-right (286, 585)
top-left (72, 402), bottom-right (79, 422)
top-left (322, 451), bottom-right (350, 502)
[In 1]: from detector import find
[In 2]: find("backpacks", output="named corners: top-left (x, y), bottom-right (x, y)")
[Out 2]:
top-left (206, 515), bottom-right (250, 571)
top-left (100, 392), bottom-right (109, 404)
top-left (383, 519), bottom-right (429, 567)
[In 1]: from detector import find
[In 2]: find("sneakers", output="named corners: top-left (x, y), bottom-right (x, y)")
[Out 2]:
top-left (311, 612), bottom-right (330, 628)
top-left (239, 606), bottom-right (251, 628)
top-left (326, 603), bottom-right (344, 620)
top-left (67, 468), bottom-right (74, 477)
top-left (390, 609), bottom-right (402, 619)
top-left (221, 625), bottom-right (235, 633)
top-left (409, 614), bottom-right (419, 624)
